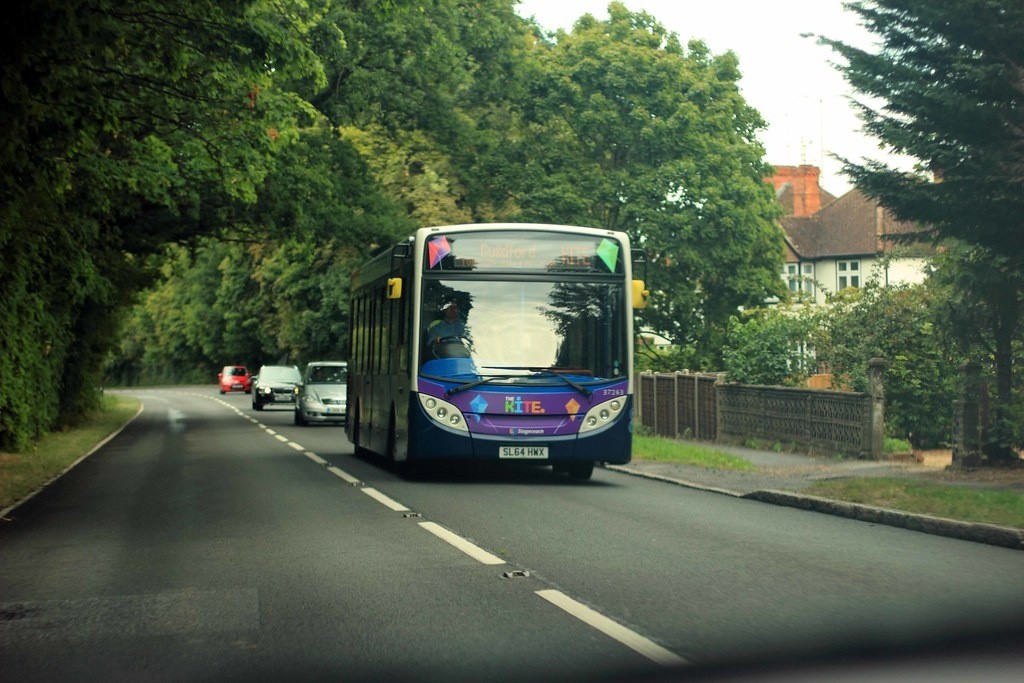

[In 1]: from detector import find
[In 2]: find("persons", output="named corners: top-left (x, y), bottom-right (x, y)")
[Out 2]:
top-left (425, 302), bottom-right (467, 352)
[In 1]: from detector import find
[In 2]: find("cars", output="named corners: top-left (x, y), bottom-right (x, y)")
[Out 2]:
top-left (218, 365), bottom-right (251, 394)
top-left (249, 366), bottom-right (302, 411)
top-left (294, 361), bottom-right (352, 424)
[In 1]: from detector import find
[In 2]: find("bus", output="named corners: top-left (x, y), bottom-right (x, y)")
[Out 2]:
top-left (341, 222), bottom-right (648, 486)
